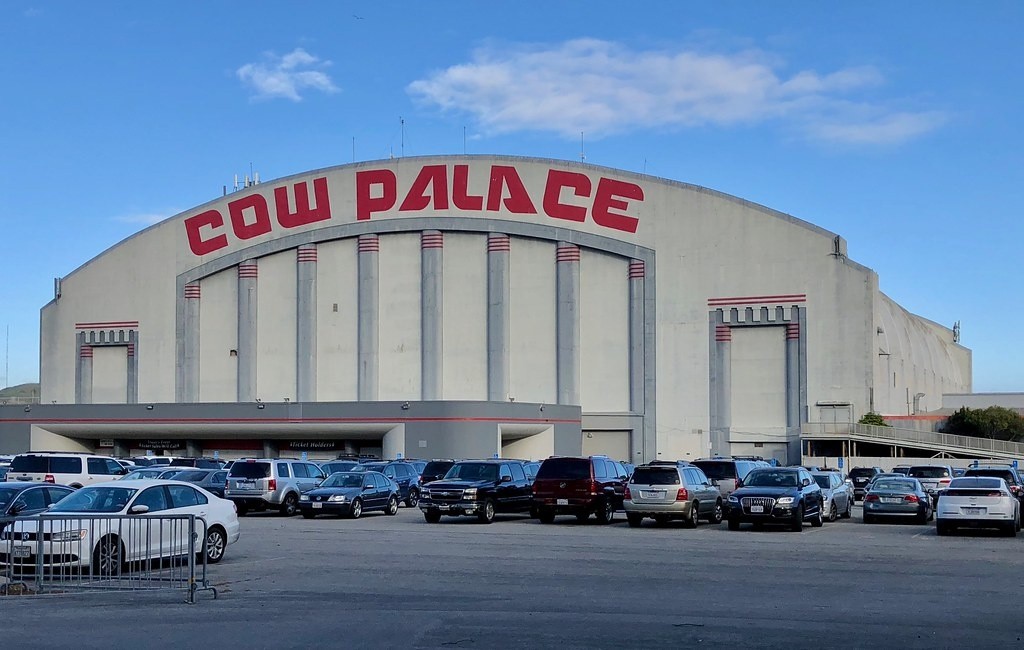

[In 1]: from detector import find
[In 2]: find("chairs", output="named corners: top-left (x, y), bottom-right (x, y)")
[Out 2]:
top-left (148, 489), bottom-right (165, 512)
top-left (176, 488), bottom-right (195, 507)
top-left (100, 489), bottom-right (130, 512)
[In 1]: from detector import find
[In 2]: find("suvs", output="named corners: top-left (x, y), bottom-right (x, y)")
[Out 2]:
top-left (5, 452), bottom-right (129, 490)
top-left (623, 459), bottom-right (724, 524)
top-left (224, 456), bottom-right (329, 517)
top-left (531, 454), bottom-right (633, 524)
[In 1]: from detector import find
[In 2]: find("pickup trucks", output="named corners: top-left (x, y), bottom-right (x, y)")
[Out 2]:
top-left (418, 458), bottom-right (536, 524)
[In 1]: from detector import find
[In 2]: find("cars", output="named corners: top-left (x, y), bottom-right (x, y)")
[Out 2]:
top-left (936, 476), bottom-right (1021, 537)
top-left (298, 470), bottom-right (402, 519)
top-left (726, 465), bottom-right (823, 532)
top-left (861, 477), bottom-right (934, 525)
top-left (168, 469), bottom-right (230, 499)
top-left (0, 452), bottom-right (1024, 522)
top-left (0, 480), bottom-right (240, 576)
top-left (119, 466), bottom-right (200, 480)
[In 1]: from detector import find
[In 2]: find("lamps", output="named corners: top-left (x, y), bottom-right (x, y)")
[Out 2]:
top-left (257, 403), bottom-right (265, 409)
top-left (399, 401), bottom-right (413, 411)
top-left (539, 404), bottom-right (548, 413)
top-left (23, 404), bottom-right (31, 413)
top-left (146, 404), bottom-right (154, 410)
